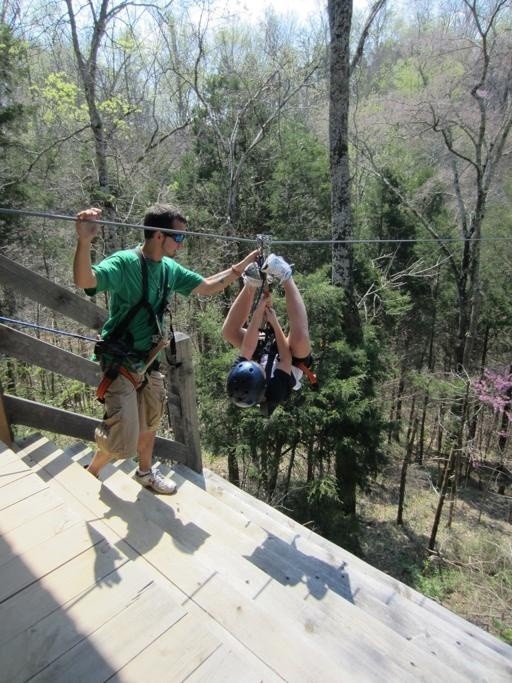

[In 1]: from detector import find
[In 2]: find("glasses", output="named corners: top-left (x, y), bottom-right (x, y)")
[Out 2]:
top-left (160, 229), bottom-right (185, 243)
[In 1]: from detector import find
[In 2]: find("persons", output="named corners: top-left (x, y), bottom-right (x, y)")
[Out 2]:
top-left (219, 251), bottom-right (316, 422)
top-left (73, 205), bottom-right (261, 496)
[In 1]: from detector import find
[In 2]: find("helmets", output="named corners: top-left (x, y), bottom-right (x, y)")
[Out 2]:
top-left (226, 360), bottom-right (266, 408)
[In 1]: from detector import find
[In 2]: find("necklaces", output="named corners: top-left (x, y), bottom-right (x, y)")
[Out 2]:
top-left (141, 250), bottom-right (166, 298)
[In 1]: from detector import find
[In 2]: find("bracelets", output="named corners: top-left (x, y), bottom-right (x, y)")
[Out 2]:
top-left (231, 263), bottom-right (243, 277)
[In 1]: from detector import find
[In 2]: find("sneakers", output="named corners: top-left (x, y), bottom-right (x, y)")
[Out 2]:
top-left (241, 253), bottom-right (292, 286)
top-left (134, 466), bottom-right (177, 494)
